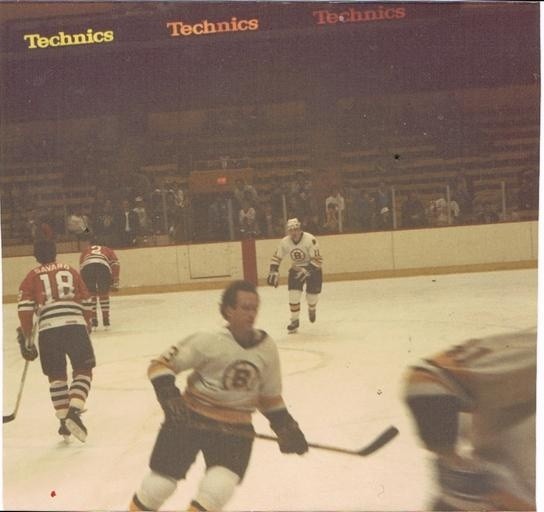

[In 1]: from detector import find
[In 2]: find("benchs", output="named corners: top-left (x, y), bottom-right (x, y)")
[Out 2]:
top-left (1, 91), bottom-right (539, 252)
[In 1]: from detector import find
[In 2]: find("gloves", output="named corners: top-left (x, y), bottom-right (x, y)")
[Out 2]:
top-left (155, 388), bottom-right (191, 427)
top-left (17, 328), bottom-right (37, 360)
top-left (110, 282), bottom-right (120, 292)
top-left (430, 452), bottom-right (500, 512)
top-left (268, 413), bottom-right (308, 455)
top-left (295, 268), bottom-right (309, 283)
top-left (267, 271), bottom-right (279, 288)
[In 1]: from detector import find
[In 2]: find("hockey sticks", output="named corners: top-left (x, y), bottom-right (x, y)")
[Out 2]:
top-left (161, 422), bottom-right (399, 456)
top-left (3, 322), bottom-right (38, 424)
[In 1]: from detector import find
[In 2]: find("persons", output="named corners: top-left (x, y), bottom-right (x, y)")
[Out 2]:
top-left (400, 324), bottom-right (535, 510)
top-left (79, 243), bottom-right (119, 329)
top-left (267, 218), bottom-right (323, 333)
top-left (14, 239), bottom-right (96, 445)
top-left (125, 281), bottom-right (307, 511)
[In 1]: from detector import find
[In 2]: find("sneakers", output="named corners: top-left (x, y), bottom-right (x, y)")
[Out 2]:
top-left (308, 310), bottom-right (317, 322)
top-left (57, 418), bottom-right (70, 435)
top-left (65, 407), bottom-right (87, 434)
top-left (91, 317), bottom-right (110, 327)
top-left (287, 320), bottom-right (300, 330)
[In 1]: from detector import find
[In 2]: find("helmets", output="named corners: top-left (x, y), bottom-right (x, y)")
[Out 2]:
top-left (285, 218), bottom-right (300, 231)
top-left (33, 239), bottom-right (56, 265)
